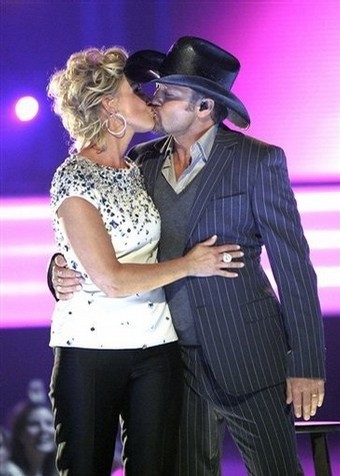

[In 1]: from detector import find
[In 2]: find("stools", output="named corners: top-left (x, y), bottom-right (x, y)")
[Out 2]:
top-left (295, 421), bottom-right (340, 476)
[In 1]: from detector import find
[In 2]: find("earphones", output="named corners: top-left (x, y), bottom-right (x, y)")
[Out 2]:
top-left (109, 100), bottom-right (116, 107)
top-left (199, 102), bottom-right (209, 111)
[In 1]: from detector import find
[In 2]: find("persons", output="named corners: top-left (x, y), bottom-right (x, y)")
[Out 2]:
top-left (45, 34), bottom-right (328, 476)
top-left (0, 45), bottom-right (244, 476)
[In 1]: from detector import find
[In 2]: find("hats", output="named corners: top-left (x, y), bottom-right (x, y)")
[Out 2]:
top-left (124, 36), bottom-right (251, 130)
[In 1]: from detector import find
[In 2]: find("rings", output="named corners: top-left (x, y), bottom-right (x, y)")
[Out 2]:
top-left (222, 252), bottom-right (232, 262)
top-left (311, 393), bottom-right (318, 398)
